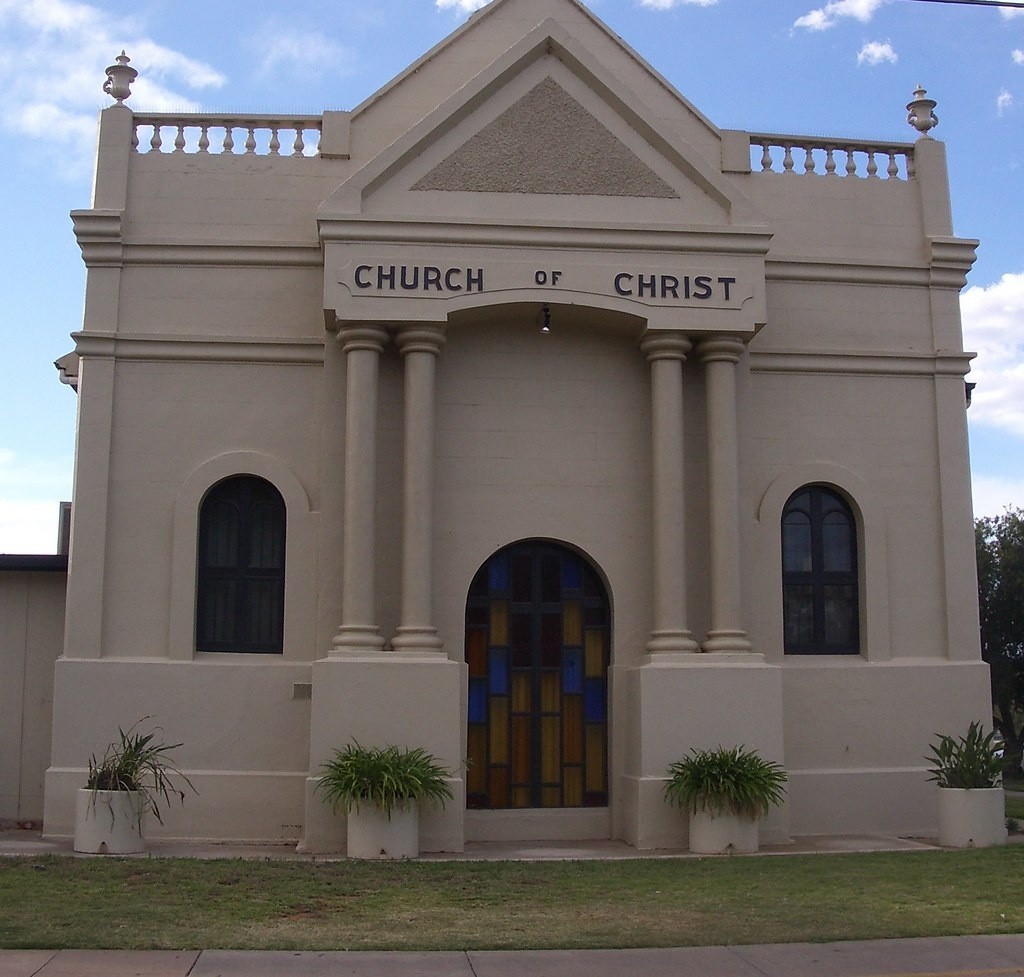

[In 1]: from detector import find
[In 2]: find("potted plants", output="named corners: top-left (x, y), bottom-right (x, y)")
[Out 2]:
top-left (659, 743), bottom-right (791, 854)
top-left (923, 721), bottom-right (1022, 849)
top-left (311, 735), bottom-right (457, 860)
top-left (71, 711), bottom-right (200, 858)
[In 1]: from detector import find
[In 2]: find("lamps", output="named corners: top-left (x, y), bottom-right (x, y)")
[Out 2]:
top-left (541, 306), bottom-right (551, 334)
